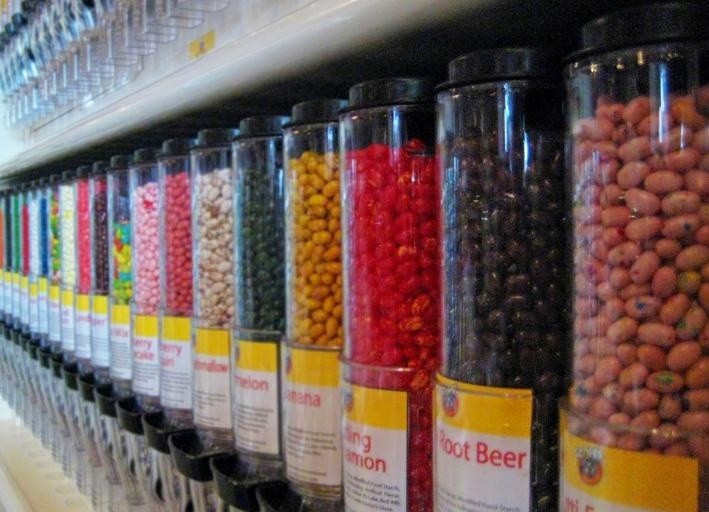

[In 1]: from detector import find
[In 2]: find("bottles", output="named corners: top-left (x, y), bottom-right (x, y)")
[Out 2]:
top-left (1, 0), bottom-right (709, 512)
